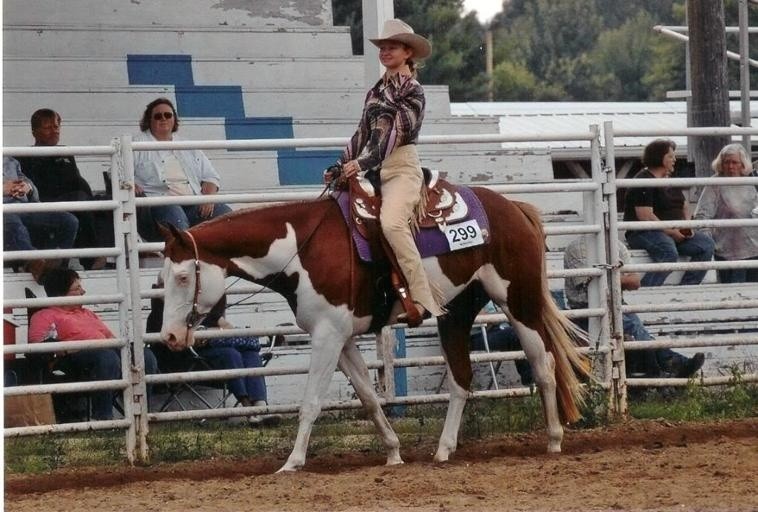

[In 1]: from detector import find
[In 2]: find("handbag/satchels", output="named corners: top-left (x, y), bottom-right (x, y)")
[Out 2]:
top-left (205, 336), bottom-right (260, 350)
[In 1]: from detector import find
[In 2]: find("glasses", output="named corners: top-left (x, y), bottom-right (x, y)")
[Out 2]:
top-left (154, 112), bottom-right (173, 120)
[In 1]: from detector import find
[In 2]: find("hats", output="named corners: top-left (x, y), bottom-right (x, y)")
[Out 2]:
top-left (368, 19), bottom-right (432, 59)
top-left (42, 266), bottom-right (74, 297)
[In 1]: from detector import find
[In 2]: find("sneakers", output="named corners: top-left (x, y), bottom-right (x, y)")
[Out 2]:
top-left (679, 354), bottom-right (704, 378)
top-left (249, 413), bottom-right (283, 426)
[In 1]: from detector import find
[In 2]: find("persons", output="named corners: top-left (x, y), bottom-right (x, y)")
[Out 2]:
top-left (562, 230), bottom-right (706, 391)
top-left (108, 97), bottom-right (233, 231)
top-left (691, 143), bottom-right (758, 283)
top-left (622, 138), bottom-right (714, 288)
top-left (28, 267), bottom-right (156, 420)
top-left (3, 155), bottom-right (80, 275)
top-left (469, 299), bottom-right (538, 386)
top-left (146, 264), bottom-right (286, 428)
top-left (322, 19), bottom-right (450, 324)
top-left (13, 109), bottom-right (117, 269)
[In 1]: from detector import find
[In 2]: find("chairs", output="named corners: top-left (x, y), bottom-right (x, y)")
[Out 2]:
top-left (25, 338), bottom-right (124, 422)
top-left (146, 283), bottom-right (276, 411)
top-left (437, 323), bottom-right (503, 394)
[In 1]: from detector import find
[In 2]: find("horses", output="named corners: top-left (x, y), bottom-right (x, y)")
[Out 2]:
top-left (157, 181), bottom-right (604, 476)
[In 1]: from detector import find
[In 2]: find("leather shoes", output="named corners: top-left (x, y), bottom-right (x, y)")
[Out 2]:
top-left (397, 301), bottom-right (431, 322)
